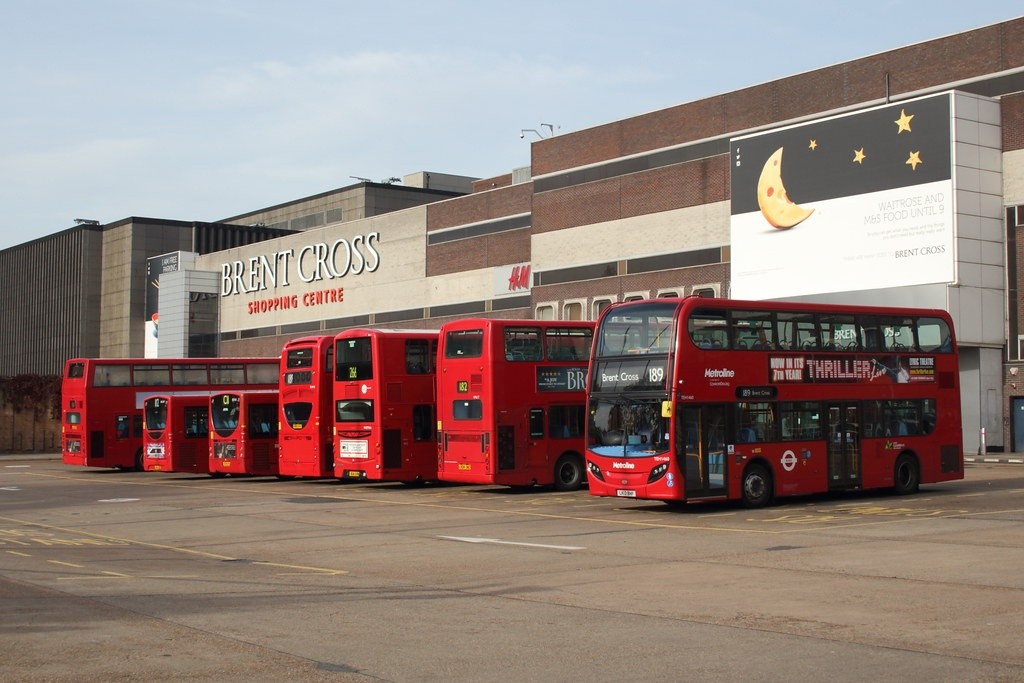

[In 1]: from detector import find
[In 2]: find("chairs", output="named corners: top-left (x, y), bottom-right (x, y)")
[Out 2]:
top-left (762, 341), bottom-right (773, 350)
top-left (192, 424), bottom-right (197, 433)
top-left (888, 420), bottom-right (908, 436)
top-left (261, 422), bottom-right (270, 432)
top-left (846, 341), bottom-right (858, 352)
top-left (700, 338), bottom-right (712, 348)
top-left (511, 350), bottom-right (525, 361)
top-left (200, 425), bottom-right (208, 433)
top-left (712, 427), bottom-right (752, 474)
top-left (228, 420), bottom-right (236, 429)
top-left (553, 346), bottom-right (575, 360)
top-left (889, 342), bottom-right (903, 352)
top-left (712, 340), bottom-right (723, 349)
top-left (251, 421), bottom-right (259, 432)
top-left (750, 340), bottom-right (763, 350)
top-left (738, 340), bottom-right (748, 350)
top-left (801, 340), bottom-right (812, 350)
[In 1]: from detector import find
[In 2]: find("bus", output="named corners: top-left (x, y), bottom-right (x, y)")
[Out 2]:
top-left (276, 335), bottom-right (341, 478)
top-left (582, 293), bottom-right (967, 509)
top-left (207, 392), bottom-right (278, 477)
top-left (61, 355), bottom-right (282, 472)
top-left (431, 320), bottom-right (774, 494)
top-left (143, 397), bottom-right (225, 477)
top-left (332, 326), bottom-right (649, 488)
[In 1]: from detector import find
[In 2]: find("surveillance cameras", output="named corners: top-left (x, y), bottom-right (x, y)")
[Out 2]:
top-left (520, 135), bottom-right (524, 138)
